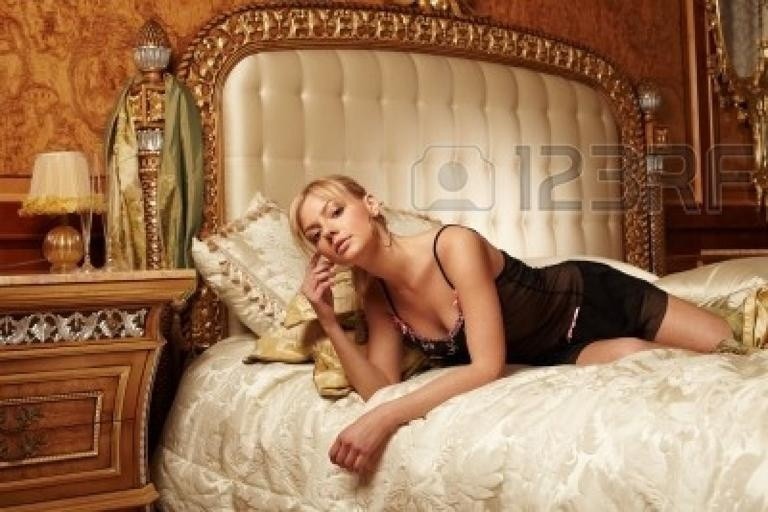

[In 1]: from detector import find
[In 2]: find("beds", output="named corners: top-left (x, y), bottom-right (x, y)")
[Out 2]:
top-left (127, 1), bottom-right (766, 511)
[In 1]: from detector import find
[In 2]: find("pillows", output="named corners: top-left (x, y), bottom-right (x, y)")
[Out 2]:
top-left (192, 195), bottom-right (324, 338)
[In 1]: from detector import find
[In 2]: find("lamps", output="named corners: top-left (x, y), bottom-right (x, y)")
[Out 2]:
top-left (18, 148), bottom-right (111, 274)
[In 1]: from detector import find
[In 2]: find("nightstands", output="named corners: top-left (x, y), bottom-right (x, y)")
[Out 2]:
top-left (0, 270), bottom-right (200, 511)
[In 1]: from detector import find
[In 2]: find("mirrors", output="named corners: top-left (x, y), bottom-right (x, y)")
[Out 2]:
top-left (704, 0), bottom-right (767, 210)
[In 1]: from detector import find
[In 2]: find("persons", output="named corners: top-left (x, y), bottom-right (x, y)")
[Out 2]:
top-left (287, 175), bottom-right (738, 469)
